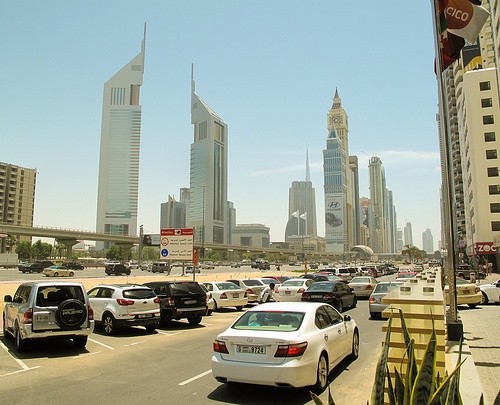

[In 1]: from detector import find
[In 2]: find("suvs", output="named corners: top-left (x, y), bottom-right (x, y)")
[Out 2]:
top-left (317, 271), bottom-right (333, 280)
top-left (62, 262), bottom-right (84, 270)
top-left (367, 268), bottom-right (377, 278)
top-left (142, 279), bottom-right (208, 326)
top-left (3, 281), bottom-right (95, 353)
top-left (251, 258), bottom-right (270, 270)
top-left (364, 263), bottom-right (398, 276)
top-left (87, 281), bottom-right (161, 336)
top-left (105, 260), bottom-right (139, 276)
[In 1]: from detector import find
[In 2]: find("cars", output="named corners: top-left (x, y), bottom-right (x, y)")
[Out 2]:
top-left (413, 263), bottom-right (424, 273)
top-left (289, 260), bottom-right (395, 269)
top-left (185, 266), bottom-right (200, 274)
top-left (395, 268), bottom-right (417, 282)
top-left (43, 266), bottom-right (74, 277)
top-left (202, 282), bottom-right (249, 311)
top-left (225, 278), bottom-right (269, 303)
top-left (202, 258), bottom-right (251, 269)
top-left (299, 274), bottom-right (322, 280)
top-left (257, 278), bottom-right (282, 289)
top-left (313, 275), bottom-right (347, 284)
top-left (272, 278), bottom-right (316, 302)
top-left (368, 281), bottom-right (404, 319)
top-left (199, 284), bottom-right (214, 316)
top-left (444, 265), bottom-right (500, 308)
top-left (346, 276), bottom-right (380, 300)
top-left (262, 277), bottom-right (290, 284)
top-left (211, 302), bottom-right (360, 392)
top-left (301, 282), bottom-right (357, 313)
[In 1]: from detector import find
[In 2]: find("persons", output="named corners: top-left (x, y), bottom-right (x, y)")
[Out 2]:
top-left (258, 283), bottom-right (276, 304)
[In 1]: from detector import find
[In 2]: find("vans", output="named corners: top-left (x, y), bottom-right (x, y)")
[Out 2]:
top-left (140, 261), bottom-right (173, 273)
top-left (345, 266), bottom-right (368, 278)
top-left (317, 268), bottom-right (351, 277)
top-left (19, 263), bottom-right (43, 274)
top-left (275, 261), bottom-right (283, 266)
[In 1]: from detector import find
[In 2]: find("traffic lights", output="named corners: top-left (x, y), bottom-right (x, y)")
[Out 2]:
top-left (143, 235), bottom-right (151, 246)
